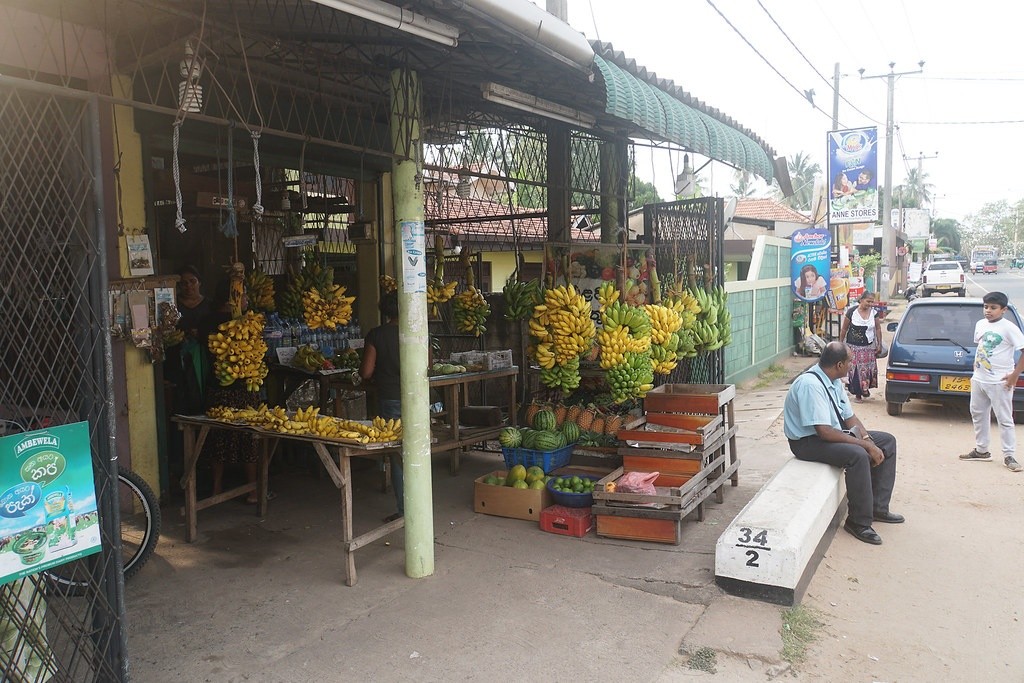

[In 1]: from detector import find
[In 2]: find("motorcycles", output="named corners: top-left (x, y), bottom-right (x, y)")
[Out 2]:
top-left (1010, 262), bottom-right (1023, 269)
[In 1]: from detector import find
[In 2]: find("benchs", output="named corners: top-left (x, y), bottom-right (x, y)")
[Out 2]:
top-left (715, 457), bottom-right (848, 606)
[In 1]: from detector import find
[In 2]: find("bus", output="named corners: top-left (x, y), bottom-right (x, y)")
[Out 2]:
top-left (970, 245), bottom-right (1000, 272)
top-left (959, 261), bottom-right (968, 272)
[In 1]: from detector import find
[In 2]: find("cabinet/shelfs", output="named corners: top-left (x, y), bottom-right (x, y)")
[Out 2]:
top-left (589, 383), bottom-right (741, 545)
top-left (265, 364), bottom-right (520, 494)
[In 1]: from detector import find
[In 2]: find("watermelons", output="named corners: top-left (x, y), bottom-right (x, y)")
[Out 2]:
top-left (499, 409), bottom-right (580, 451)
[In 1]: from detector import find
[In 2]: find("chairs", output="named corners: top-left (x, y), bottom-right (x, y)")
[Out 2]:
top-left (916, 312), bottom-right (945, 340)
top-left (968, 310), bottom-right (985, 335)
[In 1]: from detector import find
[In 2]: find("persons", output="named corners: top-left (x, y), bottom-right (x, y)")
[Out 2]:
top-left (165, 265), bottom-right (278, 505)
top-left (358, 290), bottom-right (404, 522)
top-left (959, 291), bottom-right (1024, 472)
top-left (971, 261), bottom-right (977, 272)
top-left (832, 169), bottom-right (872, 199)
top-left (840, 290), bottom-right (882, 403)
top-left (794, 264), bottom-right (827, 298)
top-left (849, 254), bottom-right (859, 273)
top-left (784, 341), bottom-right (905, 544)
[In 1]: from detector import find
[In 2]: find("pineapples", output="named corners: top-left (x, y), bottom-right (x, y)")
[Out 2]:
top-left (524, 398), bottom-right (638, 438)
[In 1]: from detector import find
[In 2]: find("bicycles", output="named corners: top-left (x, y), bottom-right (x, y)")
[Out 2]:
top-left (0, 412), bottom-right (163, 595)
top-left (971, 267), bottom-right (976, 275)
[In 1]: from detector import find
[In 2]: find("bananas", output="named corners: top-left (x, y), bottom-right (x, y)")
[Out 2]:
top-left (244, 262), bottom-right (357, 331)
top-left (163, 328), bottom-right (184, 349)
top-left (380, 274), bottom-right (492, 337)
top-left (290, 343), bottom-right (327, 371)
top-left (207, 310), bottom-right (269, 392)
top-left (205, 401), bottom-right (403, 443)
top-left (501, 276), bottom-right (732, 403)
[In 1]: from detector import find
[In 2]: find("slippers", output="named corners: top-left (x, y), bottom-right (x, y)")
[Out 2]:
top-left (247, 490), bottom-right (277, 503)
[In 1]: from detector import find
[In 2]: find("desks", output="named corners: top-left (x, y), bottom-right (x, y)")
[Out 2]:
top-left (167, 415), bottom-right (440, 588)
top-left (815, 306), bottom-right (844, 342)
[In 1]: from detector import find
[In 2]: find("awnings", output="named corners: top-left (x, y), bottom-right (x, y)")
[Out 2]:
top-left (594, 54), bottom-right (774, 186)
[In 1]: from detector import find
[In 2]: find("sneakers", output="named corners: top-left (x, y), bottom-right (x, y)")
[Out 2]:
top-left (959, 448), bottom-right (993, 462)
top-left (1003, 456), bottom-right (1022, 472)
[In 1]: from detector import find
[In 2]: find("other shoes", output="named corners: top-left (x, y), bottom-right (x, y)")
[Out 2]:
top-left (862, 391), bottom-right (870, 397)
top-left (385, 513), bottom-right (398, 522)
top-left (856, 394), bottom-right (863, 402)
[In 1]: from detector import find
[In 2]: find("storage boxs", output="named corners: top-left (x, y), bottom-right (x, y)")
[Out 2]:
top-left (874, 306), bottom-right (887, 311)
top-left (539, 505), bottom-right (592, 539)
top-left (498, 441), bottom-right (576, 476)
top-left (878, 311), bottom-right (887, 318)
top-left (874, 301), bottom-right (887, 306)
top-left (849, 287), bottom-right (864, 303)
top-left (473, 469), bottom-right (552, 522)
top-left (449, 350), bottom-right (513, 373)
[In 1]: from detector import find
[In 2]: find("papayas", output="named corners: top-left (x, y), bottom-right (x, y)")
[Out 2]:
top-left (486, 465), bottom-right (551, 489)
top-left (432, 362), bottom-right (467, 374)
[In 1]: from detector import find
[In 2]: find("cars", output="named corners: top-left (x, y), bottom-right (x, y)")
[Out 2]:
top-left (983, 259), bottom-right (997, 274)
top-left (886, 296), bottom-right (1024, 427)
top-left (976, 262), bottom-right (983, 273)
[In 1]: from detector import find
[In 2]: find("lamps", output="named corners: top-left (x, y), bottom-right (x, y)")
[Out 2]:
top-left (676, 154), bottom-right (695, 197)
top-left (479, 79), bottom-right (598, 130)
top-left (310, 0), bottom-right (460, 47)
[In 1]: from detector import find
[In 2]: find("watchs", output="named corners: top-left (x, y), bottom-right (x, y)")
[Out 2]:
top-left (863, 434), bottom-right (873, 440)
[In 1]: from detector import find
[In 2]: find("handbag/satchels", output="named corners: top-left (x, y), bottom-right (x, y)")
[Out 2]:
top-left (876, 341), bottom-right (888, 358)
top-left (846, 426), bottom-right (879, 467)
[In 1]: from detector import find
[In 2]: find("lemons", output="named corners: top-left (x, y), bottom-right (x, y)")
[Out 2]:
top-left (551, 475), bottom-right (597, 494)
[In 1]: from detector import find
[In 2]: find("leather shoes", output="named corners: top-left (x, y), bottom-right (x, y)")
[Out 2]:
top-left (872, 510), bottom-right (905, 524)
top-left (843, 520), bottom-right (882, 545)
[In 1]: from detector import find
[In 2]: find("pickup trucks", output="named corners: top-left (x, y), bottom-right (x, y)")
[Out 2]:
top-left (921, 260), bottom-right (967, 298)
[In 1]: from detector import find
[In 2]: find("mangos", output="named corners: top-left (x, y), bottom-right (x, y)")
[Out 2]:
top-left (331, 352), bottom-right (361, 369)
